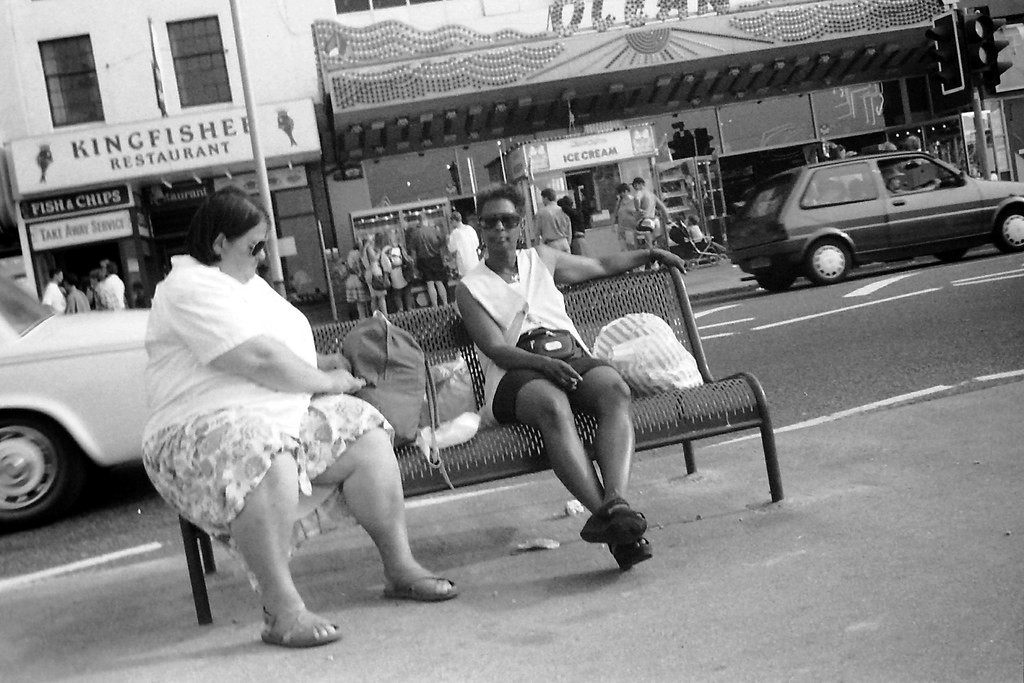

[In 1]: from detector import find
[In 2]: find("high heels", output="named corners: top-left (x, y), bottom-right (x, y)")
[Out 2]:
top-left (580, 498), bottom-right (647, 545)
top-left (607, 536), bottom-right (652, 570)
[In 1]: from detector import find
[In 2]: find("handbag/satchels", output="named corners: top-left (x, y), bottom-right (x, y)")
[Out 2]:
top-left (591, 314), bottom-right (703, 395)
top-left (399, 244), bottom-right (414, 282)
top-left (371, 272), bottom-right (391, 290)
top-left (520, 328), bottom-right (575, 361)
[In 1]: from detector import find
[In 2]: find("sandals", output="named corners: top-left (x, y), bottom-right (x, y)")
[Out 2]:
top-left (262, 605), bottom-right (340, 648)
top-left (383, 568), bottom-right (458, 601)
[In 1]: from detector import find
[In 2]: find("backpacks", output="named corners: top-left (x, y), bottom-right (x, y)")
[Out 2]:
top-left (342, 310), bottom-right (444, 469)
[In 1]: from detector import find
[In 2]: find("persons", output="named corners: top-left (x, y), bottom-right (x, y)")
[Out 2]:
top-left (687, 215), bottom-right (702, 243)
top-left (348, 231), bottom-right (415, 319)
top-left (447, 210), bottom-right (480, 278)
top-left (88, 259), bottom-right (125, 312)
top-left (614, 183), bottom-right (636, 250)
top-left (42, 268), bottom-right (67, 314)
top-left (64, 273), bottom-right (92, 314)
top-left (141, 185), bottom-right (459, 646)
top-left (532, 189), bottom-right (572, 254)
top-left (885, 167), bottom-right (941, 193)
top-left (557, 196), bottom-right (586, 255)
top-left (456, 184), bottom-right (686, 570)
top-left (401, 214), bottom-right (448, 307)
top-left (632, 177), bottom-right (672, 249)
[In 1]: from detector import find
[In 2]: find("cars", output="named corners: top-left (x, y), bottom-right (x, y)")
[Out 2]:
top-left (728, 150), bottom-right (1024, 291)
top-left (0, 276), bottom-right (152, 535)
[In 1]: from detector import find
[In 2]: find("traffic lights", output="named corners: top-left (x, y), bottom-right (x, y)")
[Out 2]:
top-left (985, 17), bottom-right (1013, 86)
top-left (959, 13), bottom-right (989, 75)
top-left (925, 11), bottom-right (973, 106)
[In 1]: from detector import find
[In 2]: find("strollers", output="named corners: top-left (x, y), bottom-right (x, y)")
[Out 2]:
top-left (667, 220), bottom-right (729, 270)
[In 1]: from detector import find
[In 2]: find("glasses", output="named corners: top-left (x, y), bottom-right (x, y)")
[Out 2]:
top-left (479, 214), bottom-right (519, 230)
top-left (230, 236), bottom-right (267, 256)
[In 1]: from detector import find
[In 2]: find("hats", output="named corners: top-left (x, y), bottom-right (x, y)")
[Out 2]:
top-left (557, 196), bottom-right (573, 208)
top-left (883, 168), bottom-right (905, 180)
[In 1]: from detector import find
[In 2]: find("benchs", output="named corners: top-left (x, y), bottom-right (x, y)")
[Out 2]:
top-left (177, 264), bottom-right (789, 626)
